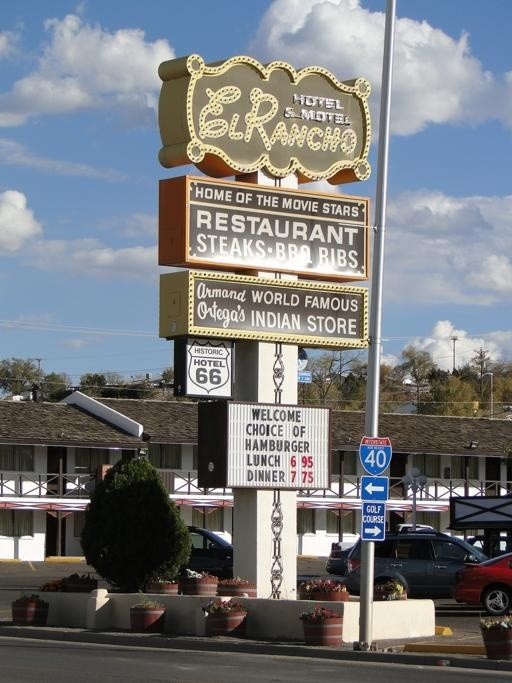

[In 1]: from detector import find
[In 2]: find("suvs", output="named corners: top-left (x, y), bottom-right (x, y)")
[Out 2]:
top-left (348, 532), bottom-right (491, 600)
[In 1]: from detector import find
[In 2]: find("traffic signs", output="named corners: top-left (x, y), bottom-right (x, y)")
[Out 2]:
top-left (359, 435), bottom-right (393, 476)
top-left (361, 503), bottom-right (387, 539)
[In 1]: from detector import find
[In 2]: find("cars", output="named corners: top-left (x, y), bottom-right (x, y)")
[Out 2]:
top-left (454, 550), bottom-right (511, 614)
top-left (174, 525), bottom-right (235, 578)
top-left (325, 541), bottom-right (353, 577)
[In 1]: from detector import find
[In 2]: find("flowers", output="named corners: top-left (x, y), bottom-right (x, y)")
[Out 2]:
top-left (302, 574), bottom-right (345, 590)
top-left (480, 615), bottom-right (512, 628)
top-left (181, 568), bottom-right (219, 585)
top-left (219, 578), bottom-right (252, 585)
top-left (299, 605), bottom-right (339, 620)
top-left (202, 596), bottom-right (242, 613)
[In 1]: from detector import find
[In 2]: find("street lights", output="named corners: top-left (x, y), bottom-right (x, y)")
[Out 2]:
top-left (449, 335), bottom-right (460, 373)
top-left (35, 357), bottom-right (43, 383)
top-left (481, 370), bottom-right (494, 419)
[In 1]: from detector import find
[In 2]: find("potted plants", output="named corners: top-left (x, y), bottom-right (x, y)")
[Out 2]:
top-left (132, 596), bottom-right (167, 633)
top-left (152, 577), bottom-right (180, 593)
top-left (9, 593), bottom-right (50, 628)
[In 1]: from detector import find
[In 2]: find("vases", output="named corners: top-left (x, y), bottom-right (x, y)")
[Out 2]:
top-left (184, 585), bottom-right (218, 595)
top-left (480, 628), bottom-right (512, 659)
top-left (218, 585), bottom-right (257, 599)
top-left (206, 612), bottom-right (249, 637)
top-left (308, 590), bottom-right (349, 602)
top-left (305, 621), bottom-right (342, 646)
